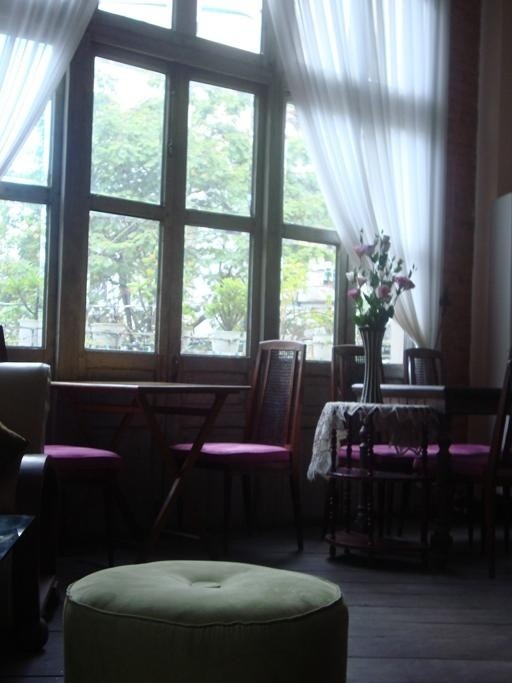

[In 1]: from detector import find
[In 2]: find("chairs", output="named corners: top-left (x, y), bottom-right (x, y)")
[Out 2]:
top-left (0, 327), bottom-right (120, 546)
top-left (326, 345), bottom-right (421, 528)
top-left (172, 341), bottom-right (307, 556)
top-left (447, 361), bottom-right (512, 576)
top-left (401, 347), bottom-right (462, 546)
top-left (1, 318), bottom-right (512, 656)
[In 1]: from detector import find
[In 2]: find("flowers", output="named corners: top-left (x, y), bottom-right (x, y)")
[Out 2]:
top-left (342, 227), bottom-right (420, 324)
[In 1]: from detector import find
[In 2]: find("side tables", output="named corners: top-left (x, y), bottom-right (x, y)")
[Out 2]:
top-left (307, 401), bottom-right (439, 572)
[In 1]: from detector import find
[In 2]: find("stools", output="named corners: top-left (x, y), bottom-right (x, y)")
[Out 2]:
top-left (59, 554), bottom-right (351, 680)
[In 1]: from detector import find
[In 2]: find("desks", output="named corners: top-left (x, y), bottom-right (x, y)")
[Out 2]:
top-left (50, 380), bottom-right (256, 551)
